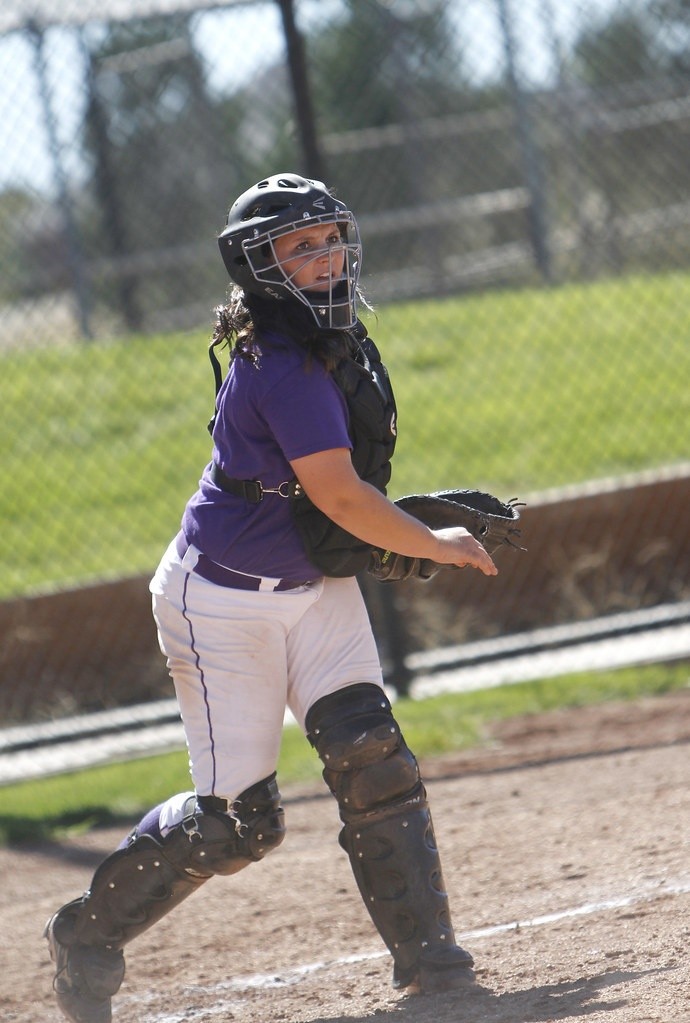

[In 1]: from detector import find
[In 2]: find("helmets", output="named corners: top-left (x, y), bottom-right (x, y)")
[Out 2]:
top-left (217, 169), bottom-right (360, 330)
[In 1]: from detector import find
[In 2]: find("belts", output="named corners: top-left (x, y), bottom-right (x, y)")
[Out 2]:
top-left (177, 535), bottom-right (312, 593)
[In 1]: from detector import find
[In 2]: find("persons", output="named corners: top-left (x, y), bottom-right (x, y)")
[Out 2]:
top-left (42, 172), bottom-right (529, 1023)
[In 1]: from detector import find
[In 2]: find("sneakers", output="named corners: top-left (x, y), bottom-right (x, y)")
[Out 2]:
top-left (407, 969), bottom-right (483, 995)
top-left (47, 908), bottom-right (112, 1022)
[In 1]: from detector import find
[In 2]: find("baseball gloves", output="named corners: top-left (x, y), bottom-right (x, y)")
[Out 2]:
top-left (366, 488), bottom-right (531, 584)
